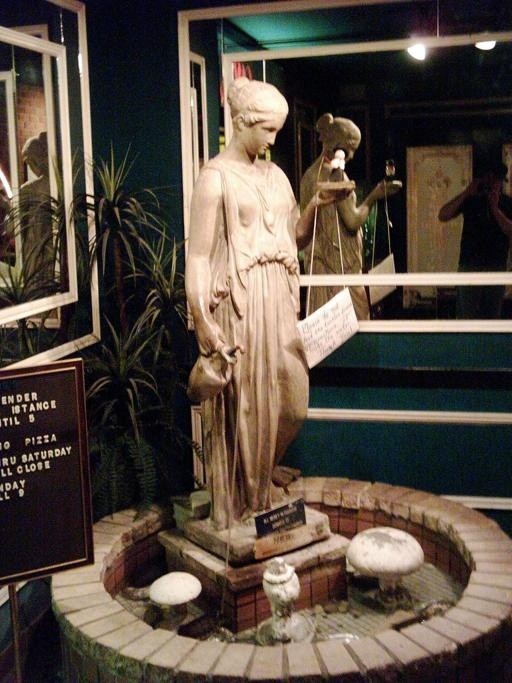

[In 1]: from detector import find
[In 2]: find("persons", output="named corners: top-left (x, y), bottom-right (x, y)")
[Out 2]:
top-left (438, 158), bottom-right (512, 321)
top-left (183, 76), bottom-right (310, 533)
top-left (298, 111), bottom-right (404, 322)
top-left (18, 131), bottom-right (59, 320)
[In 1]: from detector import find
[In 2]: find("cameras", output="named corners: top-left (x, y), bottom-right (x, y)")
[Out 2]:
top-left (478, 183), bottom-right (492, 193)
